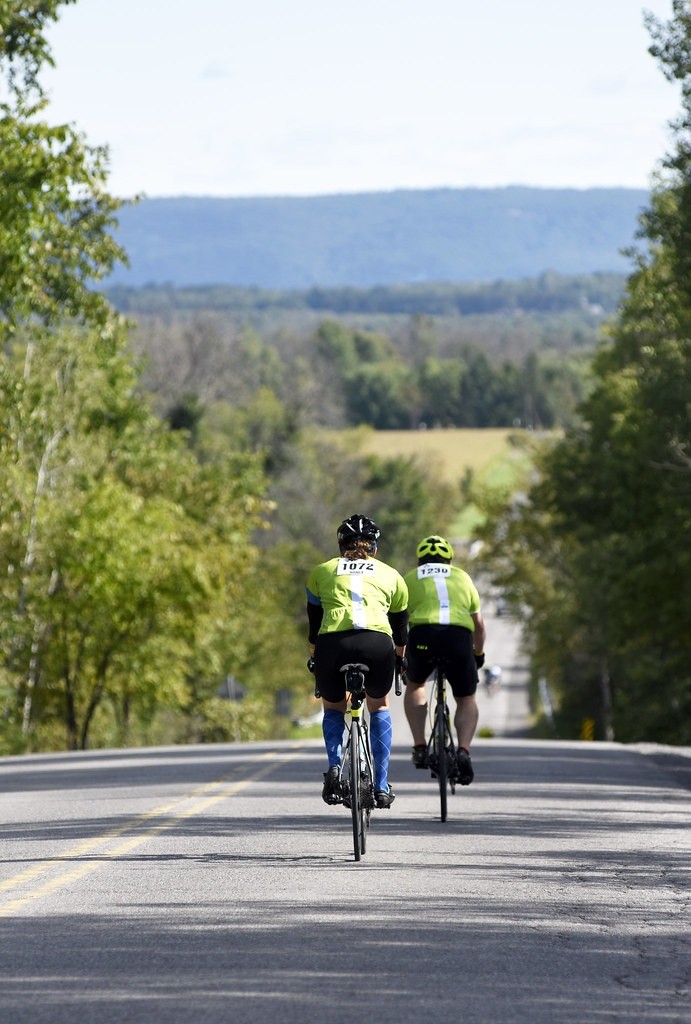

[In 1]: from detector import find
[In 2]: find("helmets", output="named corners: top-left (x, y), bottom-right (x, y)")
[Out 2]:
top-left (417, 535), bottom-right (454, 561)
top-left (337, 514), bottom-right (380, 546)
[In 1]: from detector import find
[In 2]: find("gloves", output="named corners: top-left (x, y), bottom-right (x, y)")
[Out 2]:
top-left (307, 656), bottom-right (315, 673)
top-left (473, 649), bottom-right (485, 669)
top-left (394, 650), bottom-right (408, 675)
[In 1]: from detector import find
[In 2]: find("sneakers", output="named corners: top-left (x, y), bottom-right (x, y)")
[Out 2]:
top-left (374, 783), bottom-right (396, 808)
top-left (457, 751), bottom-right (474, 785)
top-left (412, 752), bottom-right (429, 770)
top-left (322, 767), bottom-right (342, 805)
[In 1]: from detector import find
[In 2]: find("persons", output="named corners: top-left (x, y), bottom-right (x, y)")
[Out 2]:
top-left (399, 534), bottom-right (486, 785)
top-left (307, 512), bottom-right (412, 809)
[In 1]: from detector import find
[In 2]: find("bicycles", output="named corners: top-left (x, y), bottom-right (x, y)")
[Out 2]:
top-left (307, 649), bottom-right (410, 861)
top-left (401, 648), bottom-right (476, 822)
